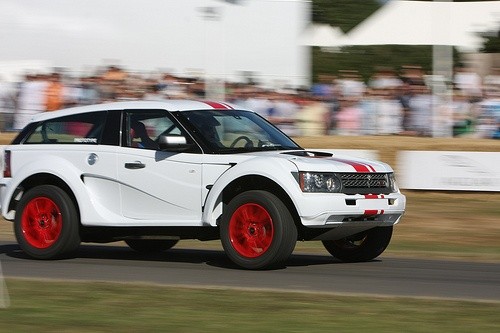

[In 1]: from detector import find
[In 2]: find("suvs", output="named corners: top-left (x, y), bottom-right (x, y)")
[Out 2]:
top-left (0, 100), bottom-right (407, 272)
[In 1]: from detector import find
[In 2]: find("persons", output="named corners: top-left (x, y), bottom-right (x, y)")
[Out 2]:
top-left (15, 65), bottom-right (499, 137)
top-left (196, 118), bottom-right (253, 150)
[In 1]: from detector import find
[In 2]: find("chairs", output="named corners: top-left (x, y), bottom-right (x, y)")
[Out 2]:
top-left (134, 121), bottom-right (160, 150)
